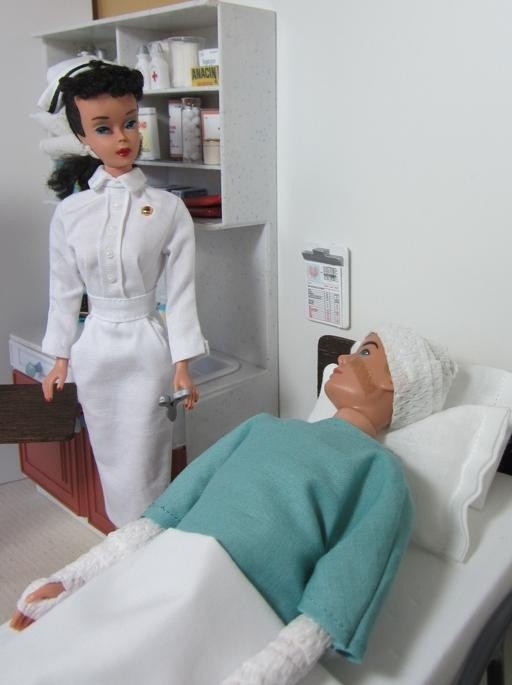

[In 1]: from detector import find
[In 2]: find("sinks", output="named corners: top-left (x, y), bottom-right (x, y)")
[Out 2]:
top-left (190, 350), bottom-right (242, 386)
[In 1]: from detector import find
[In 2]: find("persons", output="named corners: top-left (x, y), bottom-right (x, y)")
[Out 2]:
top-left (34, 54), bottom-right (213, 534)
top-left (0, 321), bottom-right (462, 685)
